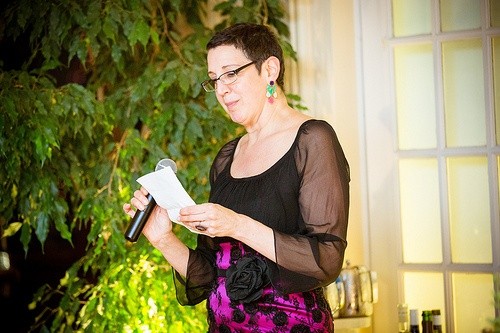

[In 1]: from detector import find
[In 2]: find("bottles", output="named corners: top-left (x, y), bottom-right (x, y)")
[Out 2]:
top-left (397, 302), bottom-right (443, 333)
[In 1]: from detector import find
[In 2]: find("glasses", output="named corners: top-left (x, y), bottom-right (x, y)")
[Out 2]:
top-left (202, 60), bottom-right (258, 93)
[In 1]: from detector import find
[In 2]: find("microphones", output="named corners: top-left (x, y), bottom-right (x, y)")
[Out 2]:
top-left (123, 158), bottom-right (178, 242)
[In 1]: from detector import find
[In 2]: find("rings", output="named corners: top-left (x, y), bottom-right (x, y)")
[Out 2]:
top-left (195, 221), bottom-right (207, 231)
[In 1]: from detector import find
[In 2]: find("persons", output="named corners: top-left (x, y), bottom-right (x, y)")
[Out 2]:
top-left (123, 22), bottom-right (350, 333)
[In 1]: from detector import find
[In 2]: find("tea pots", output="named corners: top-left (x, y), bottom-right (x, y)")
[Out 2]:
top-left (321, 259), bottom-right (377, 319)
